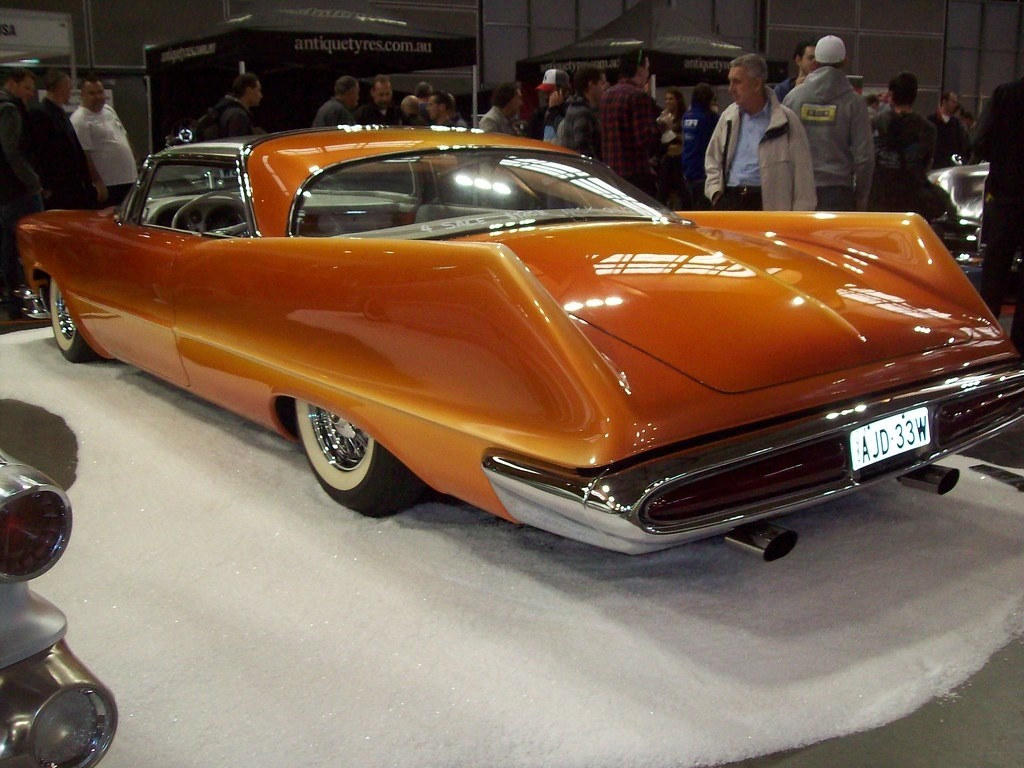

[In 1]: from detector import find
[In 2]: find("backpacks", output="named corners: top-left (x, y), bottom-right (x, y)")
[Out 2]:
top-left (191, 101), bottom-right (248, 144)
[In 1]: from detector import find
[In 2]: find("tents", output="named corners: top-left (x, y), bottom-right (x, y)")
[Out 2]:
top-left (143, 0), bottom-right (480, 153)
top-left (515, 0), bottom-right (786, 100)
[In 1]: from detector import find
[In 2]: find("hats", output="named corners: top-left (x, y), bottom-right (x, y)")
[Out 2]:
top-left (815, 35), bottom-right (846, 63)
top-left (535, 69), bottom-right (569, 91)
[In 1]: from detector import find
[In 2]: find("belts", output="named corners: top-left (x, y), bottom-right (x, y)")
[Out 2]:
top-left (726, 185), bottom-right (760, 196)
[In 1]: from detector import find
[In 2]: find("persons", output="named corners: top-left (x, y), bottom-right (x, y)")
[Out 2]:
top-left (312, 75), bottom-right (360, 127)
top-left (166, 72), bottom-right (268, 145)
top-left (400, 95), bottom-right (430, 126)
top-left (414, 82), bottom-right (434, 121)
top-left (521, 33), bottom-right (1024, 360)
top-left (426, 90), bottom-right (468, 128)
top-left (0, 66), bottom-right (139, 303)
top-left (479, 81), bottom-right (522, 137)
top-left (353, 74), bottom-right (411, 125)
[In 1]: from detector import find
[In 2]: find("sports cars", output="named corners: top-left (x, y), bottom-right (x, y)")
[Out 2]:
top-left (13, 125), bottom-right (1024, 562)
